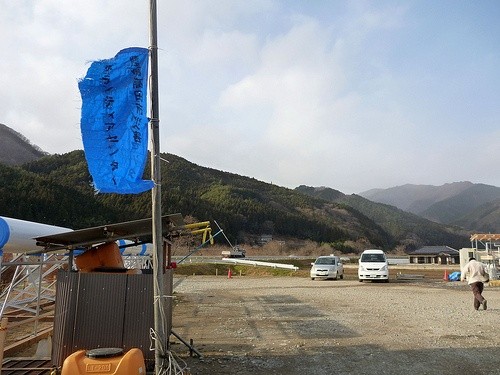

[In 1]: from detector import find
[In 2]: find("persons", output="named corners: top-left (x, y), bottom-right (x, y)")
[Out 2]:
top-left (460, 258), bottom-right (489, 310)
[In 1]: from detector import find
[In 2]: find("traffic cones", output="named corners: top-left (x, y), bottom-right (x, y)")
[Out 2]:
top-left (227, 268), bottom-right (233, 278)
top-left (443, 268), bottom-right (450, 281)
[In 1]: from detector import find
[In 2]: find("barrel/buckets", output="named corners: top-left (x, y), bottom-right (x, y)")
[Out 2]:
top-left (59, 347), bottom-right (146, 375)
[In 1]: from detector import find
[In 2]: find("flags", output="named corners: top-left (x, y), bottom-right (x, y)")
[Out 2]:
top-left (77, 45), bottom-right (155, 195)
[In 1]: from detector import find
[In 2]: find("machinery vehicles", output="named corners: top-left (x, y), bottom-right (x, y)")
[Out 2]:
top-left (221, 245), bottom-right (246, 258)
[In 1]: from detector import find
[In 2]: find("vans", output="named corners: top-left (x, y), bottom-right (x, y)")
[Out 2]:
top-left (357, 249), bottom-right (389, 285)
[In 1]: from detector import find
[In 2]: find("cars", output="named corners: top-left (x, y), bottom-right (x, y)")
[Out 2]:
top-left (309, 254), bottom-right (345, 281)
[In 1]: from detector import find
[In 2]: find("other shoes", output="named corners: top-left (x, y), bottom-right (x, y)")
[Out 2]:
top-left (483, 300), bottom-right (487, 310)
top-left (475, 307), bottom-right (478, 310)
